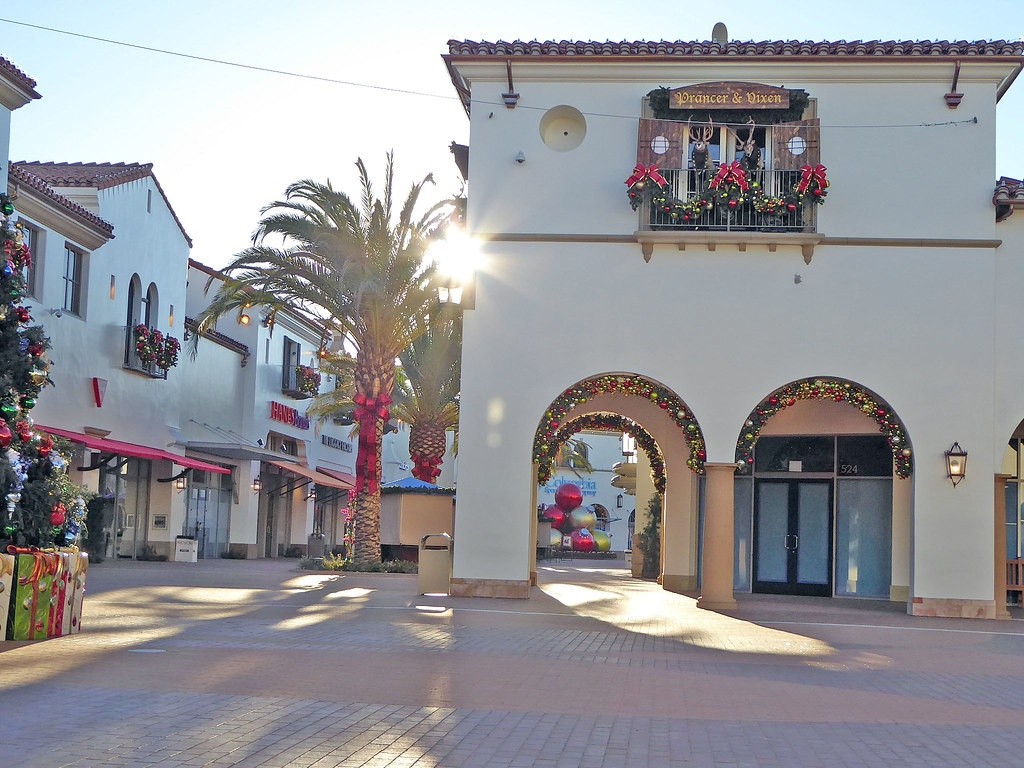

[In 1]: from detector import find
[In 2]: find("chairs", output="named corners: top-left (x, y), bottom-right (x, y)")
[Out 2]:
top-left (553, 535), bottom-right (574, 562)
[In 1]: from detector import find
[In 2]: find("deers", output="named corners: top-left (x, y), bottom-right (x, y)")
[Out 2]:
top-left (687, 113), bottom-right (714, 196)
top-left (720, 115), bottom-right (765, 186)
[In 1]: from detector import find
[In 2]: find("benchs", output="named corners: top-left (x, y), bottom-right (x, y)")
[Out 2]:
top-left (1007, 558), bottom-right (1024, 609)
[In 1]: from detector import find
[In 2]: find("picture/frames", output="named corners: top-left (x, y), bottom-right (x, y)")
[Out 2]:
top-left (152, 514), bottom-right (168, 530)
top-left (126, 513), bottom-right (140, 529)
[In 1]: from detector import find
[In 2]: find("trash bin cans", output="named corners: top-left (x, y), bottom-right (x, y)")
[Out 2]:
top-left (176, 534), bottom-right (198, 562)
top-left (308, 533), bottom-right (325, 557)
top-left (418, 532), bottom-right (454, 597)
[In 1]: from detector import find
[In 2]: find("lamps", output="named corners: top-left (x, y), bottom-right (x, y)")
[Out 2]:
top-left (310, 489), bottom-right (318, 498)
top-left (176, 470), bottom-right (188, 494)
top-left (257, 438), bottom-right (264, 448)
top-left (617, 494), bottom-right (623, 508)
top-left (254, 475), bottom-right (263, 490)
top-left (280, 444), bottom-right (287, 454)
top-left (944, 442), bottom-right (968, 487)
